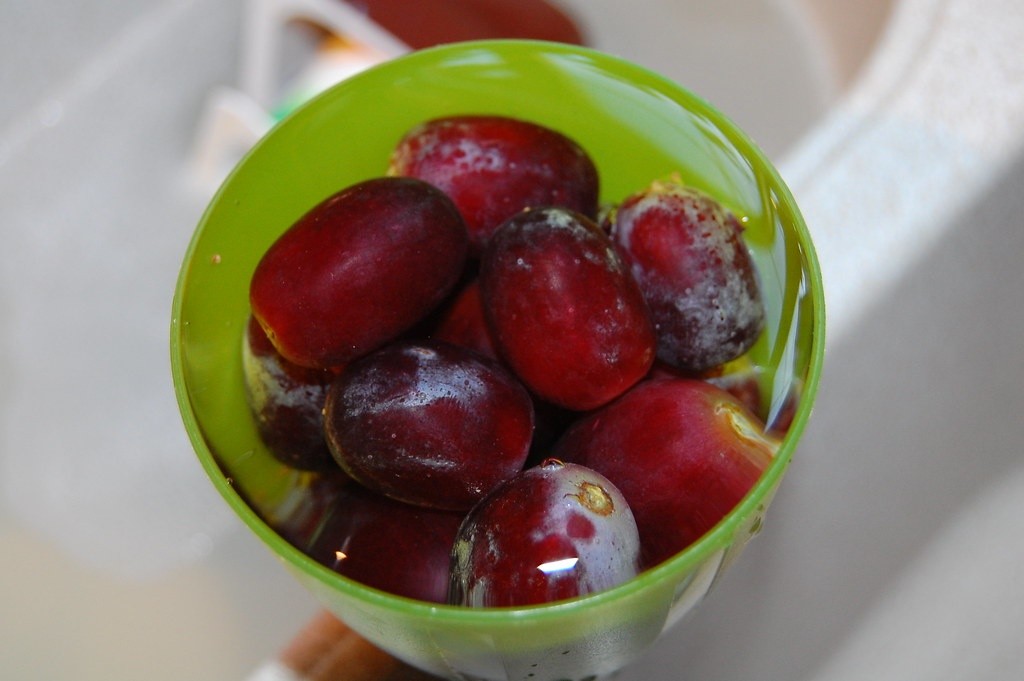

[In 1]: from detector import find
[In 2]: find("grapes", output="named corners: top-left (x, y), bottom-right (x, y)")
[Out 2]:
top-left (243, 113), bottom-right (785, 610)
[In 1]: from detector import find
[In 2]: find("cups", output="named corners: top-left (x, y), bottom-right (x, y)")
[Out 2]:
top-left (168, 38), bottom-right (825, 681)
